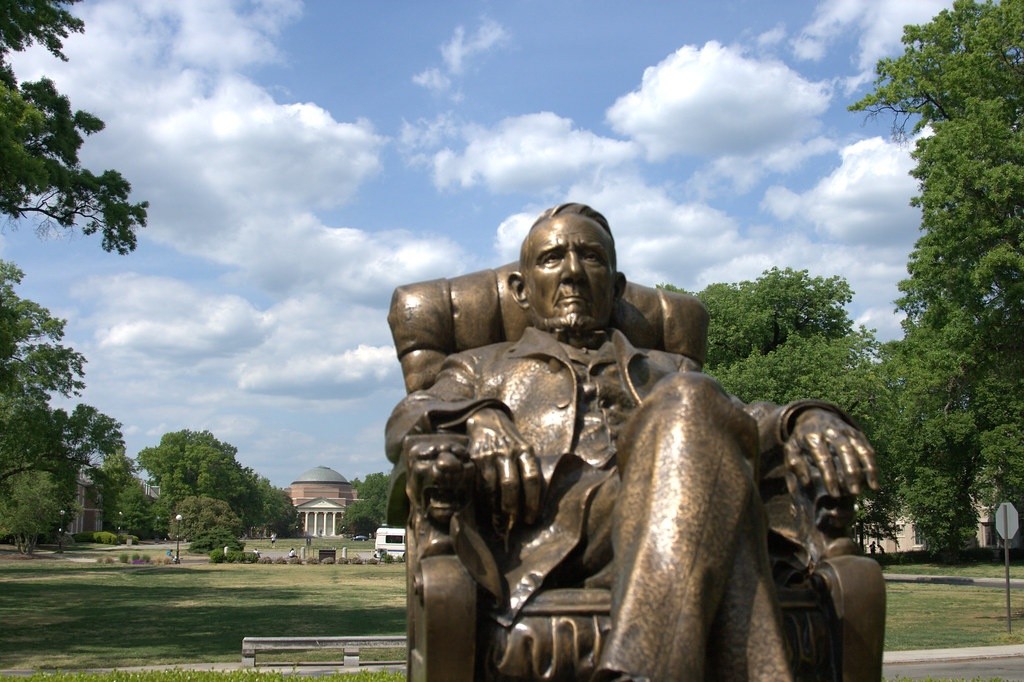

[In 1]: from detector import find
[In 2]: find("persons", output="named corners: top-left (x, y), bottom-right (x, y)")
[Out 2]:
top-left (383, 202), bottom-right (880, 682)
top-left (253, 548), bottom-right (263, 558)
top-left (166, 549), bottom-right (174, 561)
top-left (223, 543), bottom-right (231, 563)
top-left (271, 533), bottom-right (277, 548)
top-left (288, 548), bottom-right (299, 558)
top-left (869, 541), bottom-right (885, 554)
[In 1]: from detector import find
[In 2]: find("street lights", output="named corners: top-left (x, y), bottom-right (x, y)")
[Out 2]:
top-left (174, 515), bottom-right (182, 564)
top-left (116, 512), bottom-right (123, 544)
top-left (59, 510), bottom-right (65, 550)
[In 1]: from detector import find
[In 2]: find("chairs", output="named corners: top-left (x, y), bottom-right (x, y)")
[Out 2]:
top-left (388, 260), bottom-right (887, 682)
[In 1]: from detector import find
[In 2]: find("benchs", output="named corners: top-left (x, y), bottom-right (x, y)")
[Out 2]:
top-left (241, 636), bottom-right (410, 673)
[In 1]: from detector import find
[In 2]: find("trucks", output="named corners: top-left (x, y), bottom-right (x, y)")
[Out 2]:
top-left (374, 528), bottom-right (406, 561)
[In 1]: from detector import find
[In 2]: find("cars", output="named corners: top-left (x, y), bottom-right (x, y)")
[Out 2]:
top-left (352, 533), bottom-right (369, 542)
